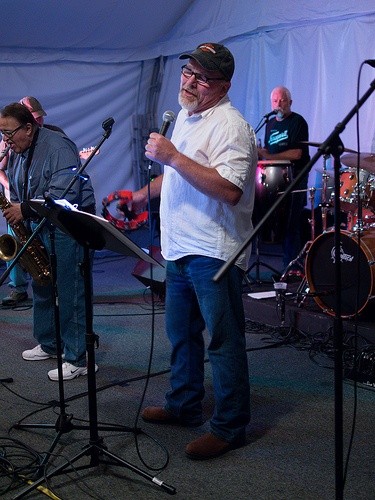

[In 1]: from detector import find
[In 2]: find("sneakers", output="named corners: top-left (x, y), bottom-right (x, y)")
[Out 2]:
top-left (143, 407), bottom-right (203, 425)
top-left (47, 360), bottom-right (99, 381)
top-left (22, 344), bottom-right (61, 360)
top-left (2, 291), bottom-right (28, 305)
top-left (185, 430), bottom-right (244, 459)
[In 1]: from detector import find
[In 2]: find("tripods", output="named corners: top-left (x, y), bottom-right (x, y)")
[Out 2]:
top-left (0, 118), bottom-right (176, 500)
top-left (240, 233), bottom-right (282, 286)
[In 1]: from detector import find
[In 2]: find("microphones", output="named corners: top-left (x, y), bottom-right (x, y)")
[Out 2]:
top-left (263, 109), bottom-right (278, 117)
top-left (364, 59), bottom-right (375, 69)
top-left (158, 110), bottom-right (175, 136)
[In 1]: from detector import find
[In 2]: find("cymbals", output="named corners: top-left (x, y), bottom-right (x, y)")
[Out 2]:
top-left (100, 190), bottom-right (148, 235)
top-left (341, 154), bottom-right (375, 176)
top-left (298, 141), bottom-right (360, 154)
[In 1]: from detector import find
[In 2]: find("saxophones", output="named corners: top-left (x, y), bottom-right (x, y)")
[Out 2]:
top-left (0, 143), bottom-right (52, 287)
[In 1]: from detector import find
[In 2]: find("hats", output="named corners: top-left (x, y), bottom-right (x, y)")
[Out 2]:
top-left (179, 43), bottom-right (235, 83)
top-left (18, 96), bottom-right (46, 119)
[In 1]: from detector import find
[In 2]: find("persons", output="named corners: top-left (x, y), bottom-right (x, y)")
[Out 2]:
top-left (255, 86), bottom-right (308, 257)
top-left (0, 95), bottom-right (99, 381)
top-left (103, 42), bottom-right (258, 460)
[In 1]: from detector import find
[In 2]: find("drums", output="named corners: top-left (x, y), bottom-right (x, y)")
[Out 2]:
top-left (327, 167), bottom-right (374, 214)
top-left (346, 206), bottom-right (375, 233)
top-left (251, 159), bottom-right (293, 224)
top-left (304, 226), bottom-right (375, 319)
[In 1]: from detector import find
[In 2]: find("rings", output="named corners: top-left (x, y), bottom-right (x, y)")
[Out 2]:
top-left (11, 217), bottom-right (14, 220)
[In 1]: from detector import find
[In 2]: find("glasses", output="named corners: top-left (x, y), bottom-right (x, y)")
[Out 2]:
top-left (1, 121), bottom-right (31, 138)
top-left (181, 64), bottom-right (227, 86)
